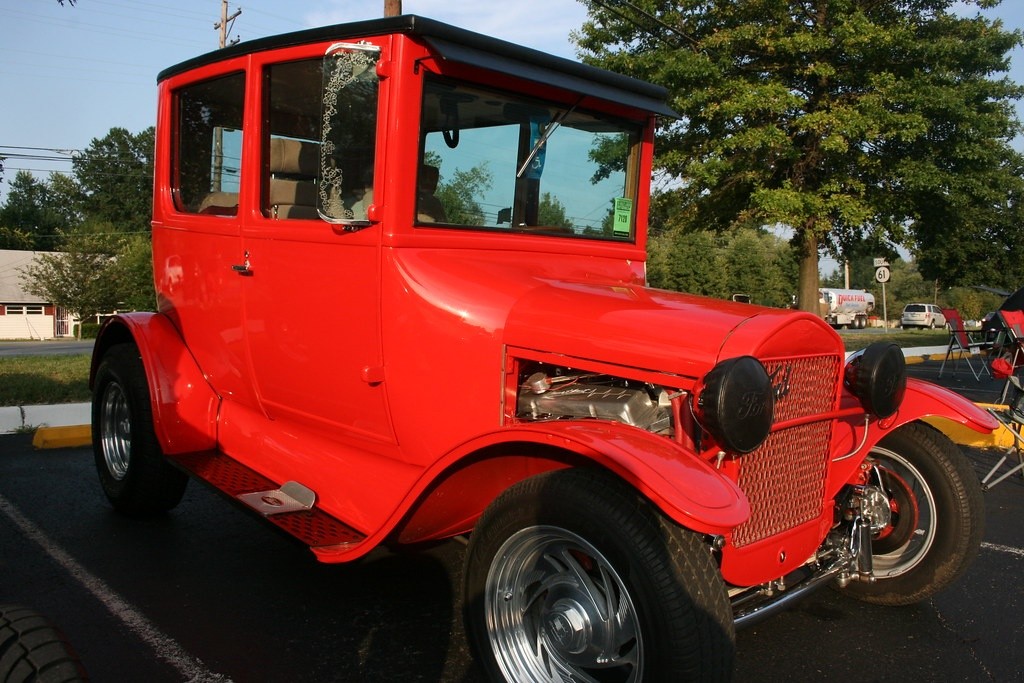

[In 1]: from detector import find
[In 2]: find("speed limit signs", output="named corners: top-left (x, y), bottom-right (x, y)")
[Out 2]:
top-left (875, 266), bottom-right (890, 283)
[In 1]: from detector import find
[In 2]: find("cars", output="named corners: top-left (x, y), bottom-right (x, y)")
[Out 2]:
top-left (982, 312), bottom-right (997, 327)
top-left (89, 12), bottom-right (1004, 682)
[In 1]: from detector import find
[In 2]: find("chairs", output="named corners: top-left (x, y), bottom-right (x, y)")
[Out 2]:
top-left (987, 310), bottom-right (1024, 406)
top-left (199, 190), bottom-right (239, 217)
top-left (363, 164), bottom-right (449, 224)
top-left (938, 307), bottom-right (996, 382)
top-left (269, 137), bottom-right (332, 221)
top-left (981, 376), bottom-right (1024, 490)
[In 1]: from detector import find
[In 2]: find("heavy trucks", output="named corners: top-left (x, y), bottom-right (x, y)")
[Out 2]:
top-left (820, 285), bottom-right (875, 329)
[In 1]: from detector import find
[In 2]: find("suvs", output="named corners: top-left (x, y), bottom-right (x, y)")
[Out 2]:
top-left (902, 302), bottom-right (950, 330)
